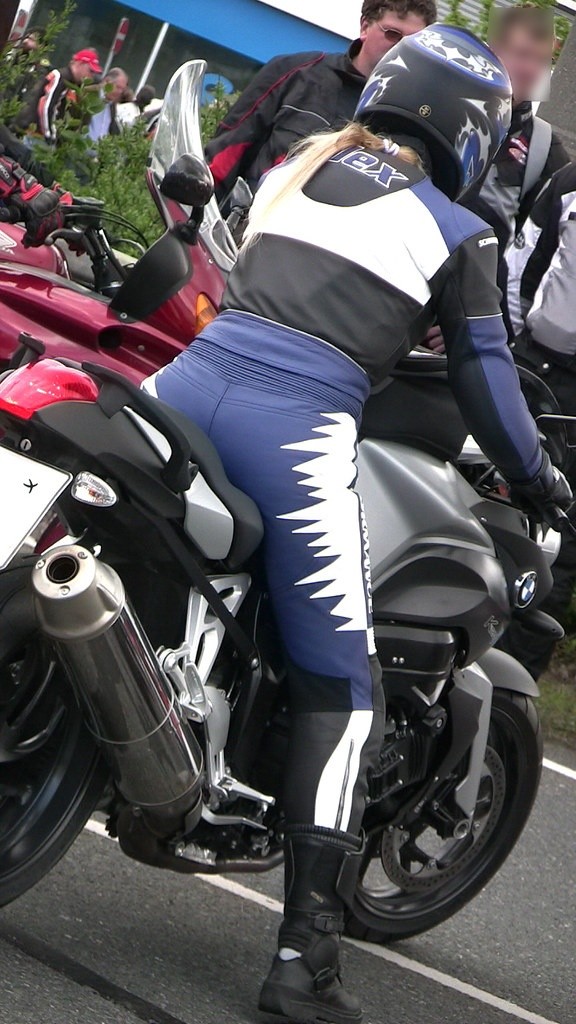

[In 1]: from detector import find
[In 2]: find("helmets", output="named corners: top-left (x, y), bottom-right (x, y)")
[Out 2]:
top-left (354, 23), bottom-right (513, 201)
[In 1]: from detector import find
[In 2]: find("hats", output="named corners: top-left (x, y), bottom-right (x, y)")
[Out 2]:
top-left (72, 48), bottom-right (103, 74)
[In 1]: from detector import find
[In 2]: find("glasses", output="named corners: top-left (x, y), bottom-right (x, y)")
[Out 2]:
top-left (377, 21), bottom-right (403, 43)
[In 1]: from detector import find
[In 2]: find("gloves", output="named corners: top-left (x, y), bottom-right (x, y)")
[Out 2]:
top-left (496, 446), bottom-right (573, 523)
top-left (50, 181), bottom-right (73, 205)
top-left (0, 156), bottom-right (65, 249)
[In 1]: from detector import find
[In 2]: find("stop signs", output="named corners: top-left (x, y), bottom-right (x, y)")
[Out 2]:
top-left (113, 18), bottom-right (129, 55)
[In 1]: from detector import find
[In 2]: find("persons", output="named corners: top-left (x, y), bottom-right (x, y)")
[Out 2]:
top-left (199, 1), bottom-right (438, 239)
top-left (0, 119), bottom-right (74, 249)
top-left (363, 2), bottom-right (571, 458)
top-left (134, 21), bottom-right (576, 1022)
top-left (9, 27), bottom-right (166, 156)
top-left (497, 160), bottom-right (575, 643)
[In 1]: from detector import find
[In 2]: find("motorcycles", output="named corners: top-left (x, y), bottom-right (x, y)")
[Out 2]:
top-left (1, 330), bottom-right (576, 945)
top-left (1, 58), bottom-right (254, 389)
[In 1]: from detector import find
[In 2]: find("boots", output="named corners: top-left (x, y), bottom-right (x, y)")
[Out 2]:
top-left (257, 826), bottom-right (364, 1024)
